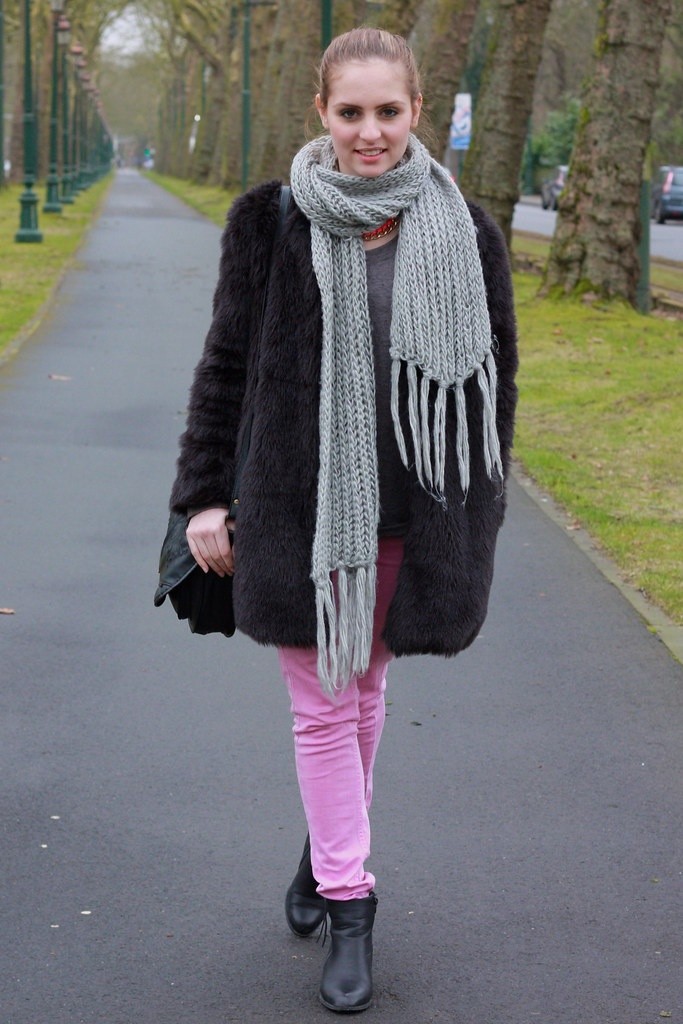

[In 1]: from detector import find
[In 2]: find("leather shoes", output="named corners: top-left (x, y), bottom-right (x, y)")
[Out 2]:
top-left (285, 829), bottom-right (327, 939)
top-left (320, 892), bottom-right (375, 1012)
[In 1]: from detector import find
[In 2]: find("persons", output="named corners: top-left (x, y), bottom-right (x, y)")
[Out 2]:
top-left (151, 26), bottom-right (523, 1015)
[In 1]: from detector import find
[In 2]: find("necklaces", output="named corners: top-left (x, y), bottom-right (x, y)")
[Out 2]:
top-left (358, 217), bottom-right (406, 243)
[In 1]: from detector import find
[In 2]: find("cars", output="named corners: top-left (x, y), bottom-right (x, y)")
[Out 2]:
top-left (540, 165), bottom-right (571, 211)
top-left (649, 165), bottom-right (683, 223)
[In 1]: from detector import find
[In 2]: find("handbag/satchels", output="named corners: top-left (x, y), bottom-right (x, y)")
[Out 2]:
top-left (154, 503), bottom-right (239, 642)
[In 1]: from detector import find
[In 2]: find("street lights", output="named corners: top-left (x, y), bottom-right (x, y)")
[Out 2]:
top-left (42, 1), bottom-right (113, 215)
